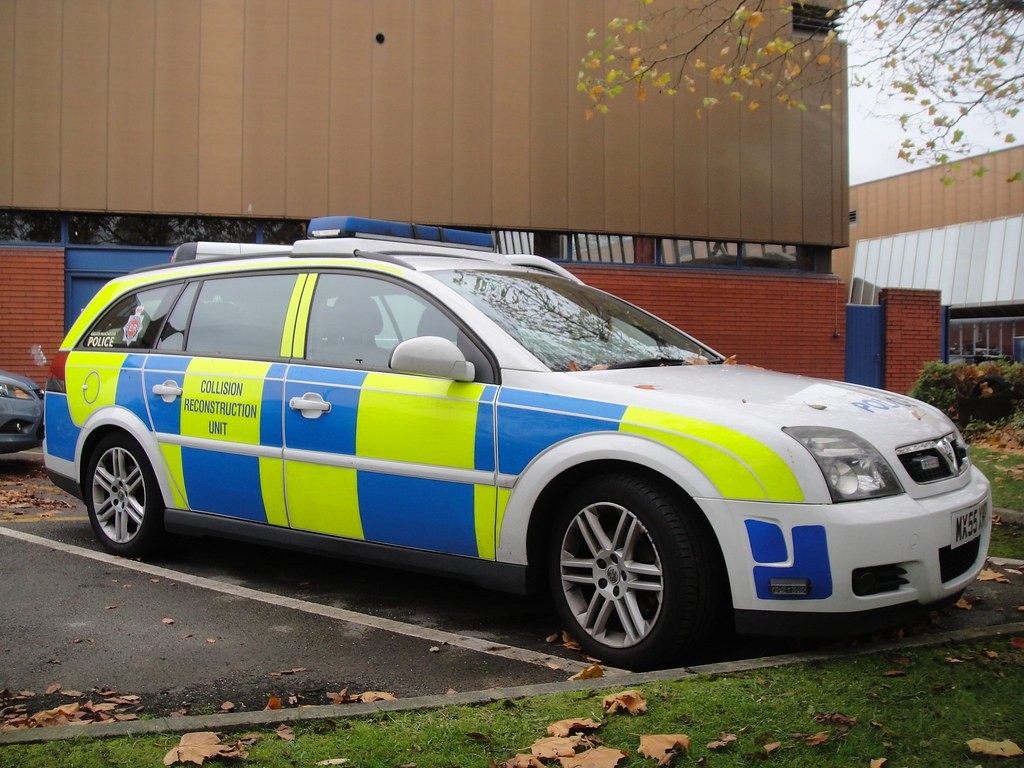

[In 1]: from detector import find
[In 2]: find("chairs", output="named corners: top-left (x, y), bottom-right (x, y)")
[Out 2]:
top-left (417, 305), bottom-right (460, 343)
top-left (323, 293), bottom-right (390, 369)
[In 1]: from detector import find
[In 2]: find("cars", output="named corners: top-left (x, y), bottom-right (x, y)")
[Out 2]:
top-left (40, 212), bottom-right (993, 670)
